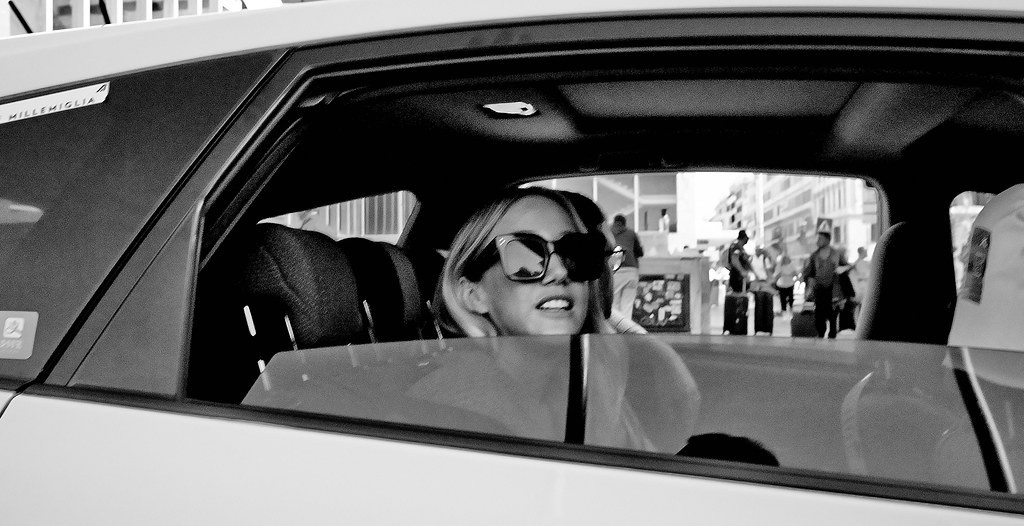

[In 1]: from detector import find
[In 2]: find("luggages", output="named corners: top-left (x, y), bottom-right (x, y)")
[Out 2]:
top-left (754, 285), bottom-right (773, 336)
top-left (723, 278), bottom-right (748, 335)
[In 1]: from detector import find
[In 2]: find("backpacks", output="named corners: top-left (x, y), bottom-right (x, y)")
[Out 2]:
top-left (717, 242), bottom-right (746, 272)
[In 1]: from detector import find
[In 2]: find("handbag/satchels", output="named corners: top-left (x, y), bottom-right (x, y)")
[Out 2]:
top-left (791, 310), bottom-right (819, 336)
top-left (839, 255), bottom-right (856, 298)
top-left (804, 278), bottom-right (816, 302)
top-left (803, 256), bottom-right (816, 283)
top-left (838, 298), bottom-right (857, 331)
top-left (633, 231), bottom-right (643, 258)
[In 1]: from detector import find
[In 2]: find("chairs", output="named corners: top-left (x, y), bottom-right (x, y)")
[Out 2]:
top-left (841, 219), bottom-right (950, 480)
top-left (225, 222), bottom-right (363, 412)
top-left (334, 237), bottom-right (435, 364)
top-left (934, 183), bottom-right (1024, 493)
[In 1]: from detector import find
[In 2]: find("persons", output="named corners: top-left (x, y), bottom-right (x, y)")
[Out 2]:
top-left (722, 230), bottom-right (871, 339)
top-left (403, 187), bottom-right (700, 456)
top-left (607, 214), bottom-right (648, 336)
top-left (658, 209), bottom-right (672, 234)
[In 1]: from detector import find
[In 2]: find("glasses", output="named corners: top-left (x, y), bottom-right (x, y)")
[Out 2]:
top-left (464, 231), bottom-right (616, 283)
top-left (605, 244), bottom-right (626, 274)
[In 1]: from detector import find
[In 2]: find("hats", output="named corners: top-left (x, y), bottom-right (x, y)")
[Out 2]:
top-left (737, 230), bottom-right (747, 238)
